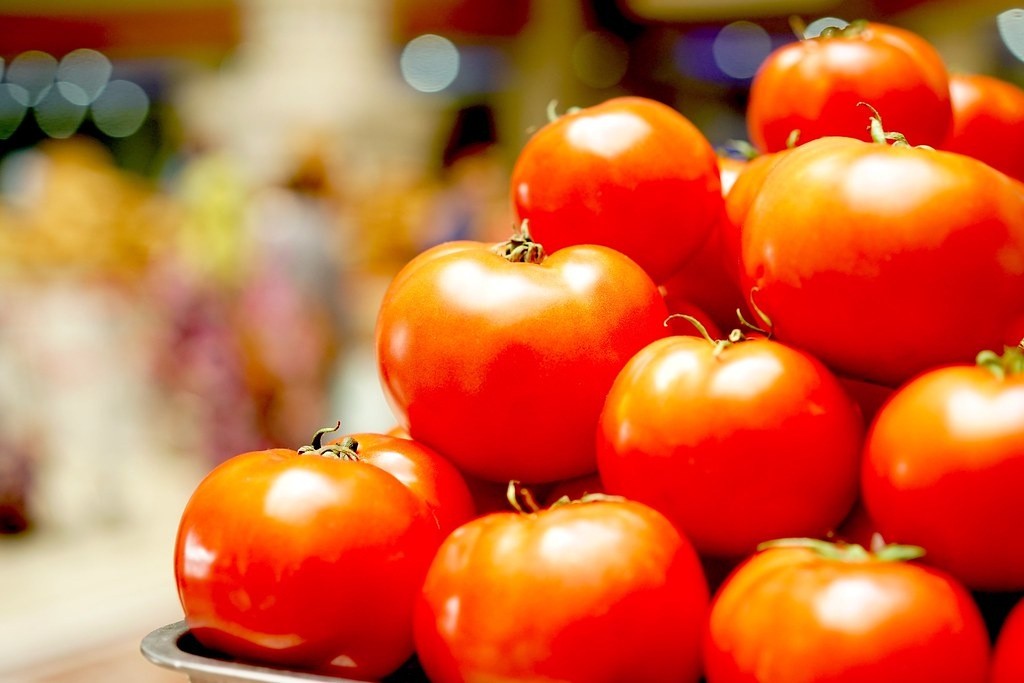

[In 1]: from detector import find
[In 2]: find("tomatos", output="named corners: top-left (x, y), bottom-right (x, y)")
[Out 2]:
top-left (172, 20), bottom-right (1024, 683)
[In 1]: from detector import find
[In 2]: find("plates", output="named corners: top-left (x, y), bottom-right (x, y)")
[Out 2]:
top-left (141, 622), bottom-right (364, 683)
